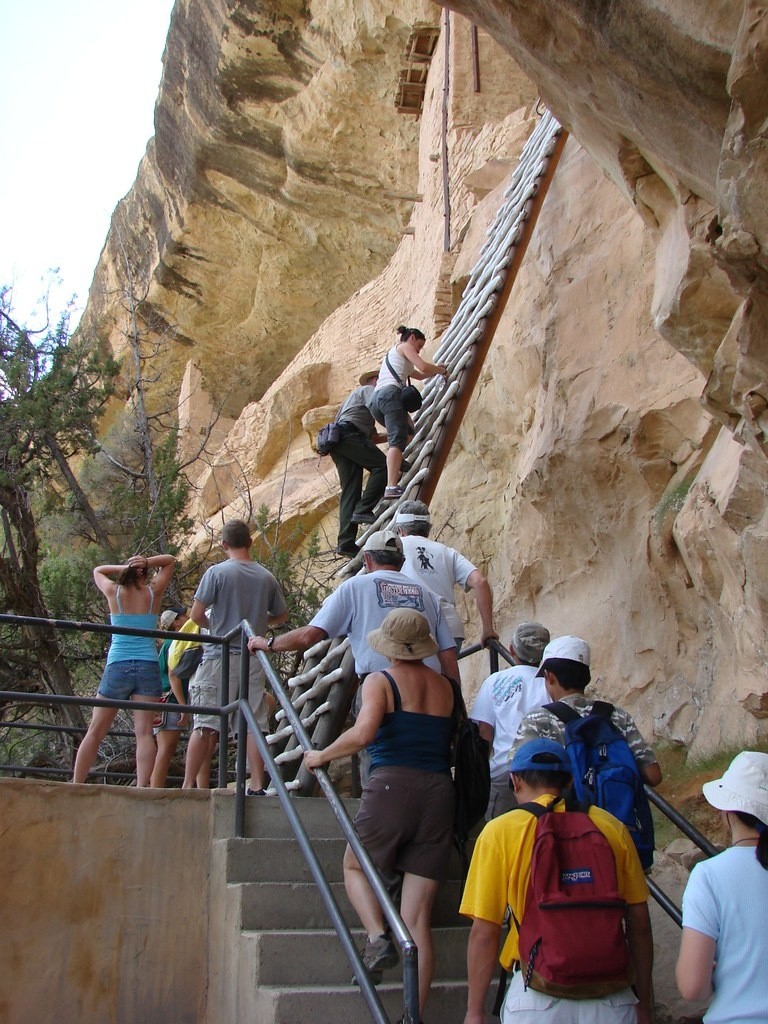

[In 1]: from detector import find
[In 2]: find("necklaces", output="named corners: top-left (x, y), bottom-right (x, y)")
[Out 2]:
top-left (733, 837), bottom-right (759, 846)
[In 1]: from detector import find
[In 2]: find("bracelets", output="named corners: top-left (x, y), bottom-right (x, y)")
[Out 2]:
top-left (145, 558), bottom-right (148, 568)
top-left (443, 370), bottom-right (447, 375)
top-left (267, 637), bottom-right (274, 652)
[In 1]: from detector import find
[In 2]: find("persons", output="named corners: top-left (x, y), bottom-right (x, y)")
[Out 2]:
top-left (368, 325), bottom-right (450, 500)
top-left (248, 531), bottom-right (462, 904)
top-left (395, 500), bottom-right (500, 656)
top-left (470, 620), bottom-right (553, 823)
top-left (674, 751), bottom-right (768, 1024)
top-left (71, 555), bottom-right (174, 788)
top-left (329, 370), bottom-right (387, 558)
top-left (459, 738), bottom-right (654, 1024)
top-left (150, 607), bottom-right (218, 787)
top-left (304, 608), bottom-right (467, 1024)
top-left (190, 519), bottom-right (288, 796)
top-left (507, 633), bottom-right (662, 789)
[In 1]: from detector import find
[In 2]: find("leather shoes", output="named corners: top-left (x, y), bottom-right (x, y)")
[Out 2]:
top-left (351, 511), bottom-right (377, 523)
top-left (338, 543), bottom-right (360, 557)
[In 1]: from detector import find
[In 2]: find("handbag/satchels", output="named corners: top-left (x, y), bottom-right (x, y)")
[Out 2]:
top-left (316, 422), bottom-right (340, 456)
top-left (445, 673), bottom-right (495, 835)
top-left (172, 647), bottom-right (205, 679)
top-left (152, 691), bottom-right (172, 728)
top-left (402, 384), bottom-right (422, 412)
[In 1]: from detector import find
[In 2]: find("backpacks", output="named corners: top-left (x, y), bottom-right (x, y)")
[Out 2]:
top-left (515, 790), bottom-right (635, 996)
top-left (544, 699), bottom-right (656, 870)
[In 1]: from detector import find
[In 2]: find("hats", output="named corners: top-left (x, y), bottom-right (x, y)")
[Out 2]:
top-left (367, 608), bottom-right (439, 660)
top-left (359, 366), bottom-right (380, 386)
top-left (535, 635), bottom-right (590, 676)
top-left (509, 739), bottom-right (571, 791)
top-left (363, 530), bottom-right (404, 554)
top-left (514, 621), bottom-right (550, 662)
top-left (703, 750), bottom-right (768, 824)
top-left (161, 607), bottom-right (187, 629)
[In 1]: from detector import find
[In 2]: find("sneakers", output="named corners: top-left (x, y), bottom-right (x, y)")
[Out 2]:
top-left (351, 934), bottom-right (400, 985)
top-left (385, 485), bottom-right (406, 498)
